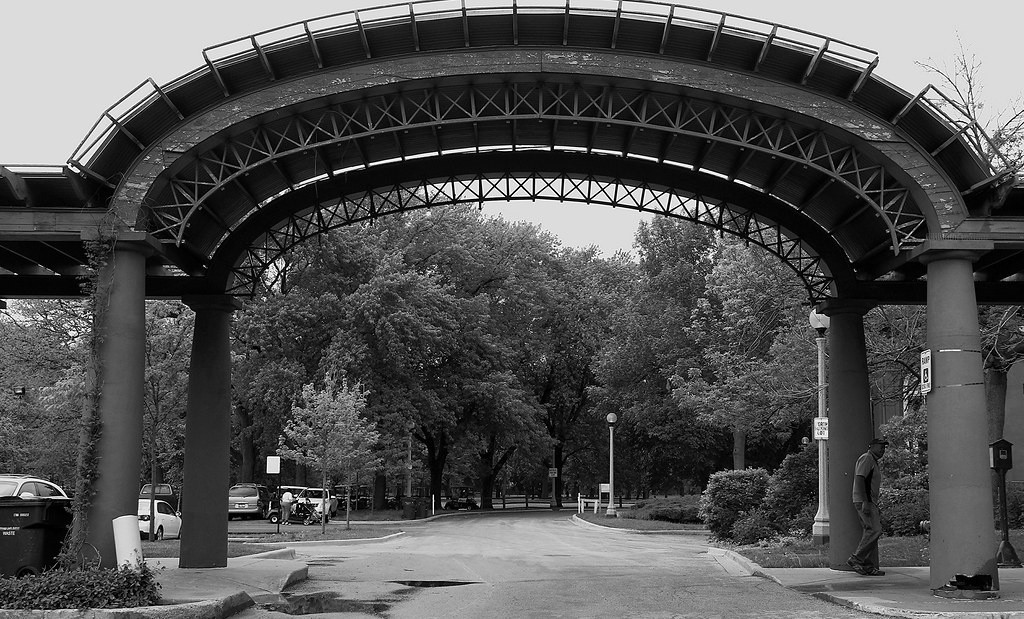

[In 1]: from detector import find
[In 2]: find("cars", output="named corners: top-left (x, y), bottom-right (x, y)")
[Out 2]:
top-left (139, 484), bottom-right (178, 513)
top-left (228, 482), bottom-right (270, 521)
top-left (138, 499), bottom-right (182, 541)
top-left (266, 486), bottom-right (338, 524)
top-left (0, 478), bottom-right (69, 499)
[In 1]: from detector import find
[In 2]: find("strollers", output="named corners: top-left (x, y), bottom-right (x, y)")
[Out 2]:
top-left (302, 498), bottom-right (322, 525)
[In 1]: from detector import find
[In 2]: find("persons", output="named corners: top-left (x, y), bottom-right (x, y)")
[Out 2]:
top-left (269, 488), bottom-right (296, 525)
top-left (846, 439), bottom-right (890, 576)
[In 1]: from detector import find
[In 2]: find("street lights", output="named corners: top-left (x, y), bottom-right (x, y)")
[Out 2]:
top-left (607, 413), bottom-right (617, 518)
top-left (406, 421), bottom-right (415, 497)
top-left (809, 309), bottom-right (830, 545)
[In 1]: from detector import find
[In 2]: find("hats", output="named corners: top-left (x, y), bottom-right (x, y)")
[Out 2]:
top-left (868, 438), bottom-right (889, 447)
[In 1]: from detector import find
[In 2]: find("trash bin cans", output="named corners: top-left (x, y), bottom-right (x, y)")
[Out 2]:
top-left (0, 495), bottom-right (74, 579)
top-left (416, 497), bottom-right (430, 519)
top-left (400, 497), bottom-right (417, 520)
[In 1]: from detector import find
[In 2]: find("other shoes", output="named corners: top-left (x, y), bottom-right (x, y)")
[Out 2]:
top-left (865, 567), bottom-right (885, 576)
top-left (285, 522), bottom-right (289, 525)
top-left (846, 560), bottom-right (867, 575)
top-left (282, 522), bottom-right (285, 525)
